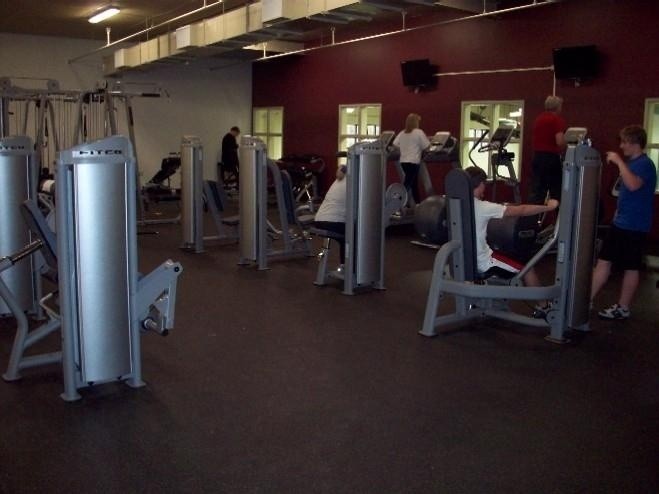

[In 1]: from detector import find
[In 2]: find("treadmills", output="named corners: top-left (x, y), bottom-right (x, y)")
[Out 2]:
top-left (265, 155), bottom-right (325, 206)
top-left (530, 127), bottom-right (591, 254)
top-left (387, 132), bottom-right (457, 228)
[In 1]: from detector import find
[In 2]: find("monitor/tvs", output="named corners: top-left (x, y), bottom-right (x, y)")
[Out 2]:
top-left (429, 132), bottom-right (450, 152)
top-left (377, 131), bottom-right (395, 149)
top-left (401, 58), bottom-right (433, 86)
top-left (564, 127), bottom-right (587, 144)
top-left (553, 44), bottom-right (599, 80)
top-left (488, 126), bottom-right (514, 147)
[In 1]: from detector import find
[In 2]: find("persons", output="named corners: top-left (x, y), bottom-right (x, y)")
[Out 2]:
top-left (588, 125), bottom-right (657, 320)
top-left (520, 96), bottom-right (570, 230)
top-left (314, 164), bottom-right (347, 272)
top-left (464, 165), bottom-right (560, 313)
top-left (393, 112), bottom-right (431, 218)
top-left (220, 125), bottom-right (241, 191)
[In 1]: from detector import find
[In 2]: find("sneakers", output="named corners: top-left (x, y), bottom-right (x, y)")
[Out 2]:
top-left (597, 302), bottom-right (630, 320)
top-left (535, 302), bottom-right (553, 314)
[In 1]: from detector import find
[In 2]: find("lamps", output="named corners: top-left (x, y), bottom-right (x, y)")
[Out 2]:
top-left (87, 7), bottom-right (120, 25)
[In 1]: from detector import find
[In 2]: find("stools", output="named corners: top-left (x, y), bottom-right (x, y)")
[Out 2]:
top-left (309, 228), bottom-right (341, 286)
